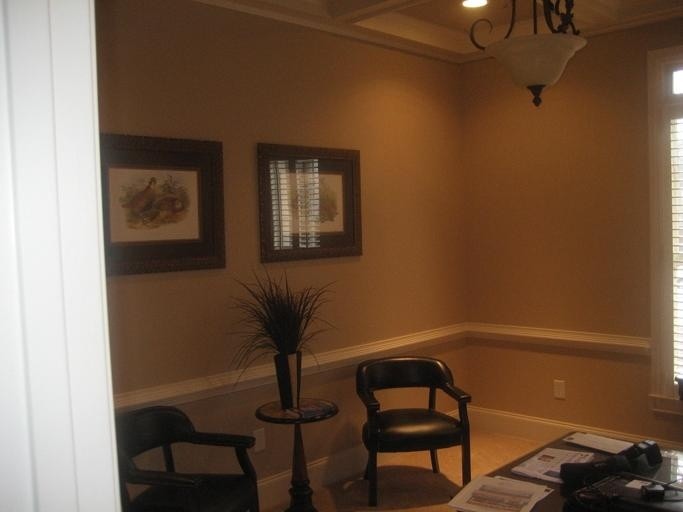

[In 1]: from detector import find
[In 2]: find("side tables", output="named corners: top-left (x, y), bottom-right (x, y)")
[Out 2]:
top-left (254, 397), bottom-right (340, 511)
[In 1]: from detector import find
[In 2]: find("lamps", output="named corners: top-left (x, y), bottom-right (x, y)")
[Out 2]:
top-left (468, 0), bottom-right (589, 108)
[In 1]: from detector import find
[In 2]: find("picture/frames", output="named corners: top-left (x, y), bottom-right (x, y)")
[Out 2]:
top-left (98, 130), bottom-right (363, 275)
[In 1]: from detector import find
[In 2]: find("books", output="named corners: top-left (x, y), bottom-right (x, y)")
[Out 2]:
top-left (563, 431), bottom-right (635, 455)
top-left (511, 447), bottom-right (595, 485)
top-left (448, 473), bottom-right (553, 511)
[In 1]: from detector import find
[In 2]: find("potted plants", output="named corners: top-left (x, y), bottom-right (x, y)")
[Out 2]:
top-left (223, 261), bottom-right (339, 410)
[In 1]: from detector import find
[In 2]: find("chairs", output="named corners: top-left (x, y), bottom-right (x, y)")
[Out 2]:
top-left (354, 355), bottom-right (473, 508)
top-left (114, 404), bottom-right (260, 512)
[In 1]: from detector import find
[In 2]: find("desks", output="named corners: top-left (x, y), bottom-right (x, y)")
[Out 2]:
top-left (448, 430), bottom-right (681, 512)
